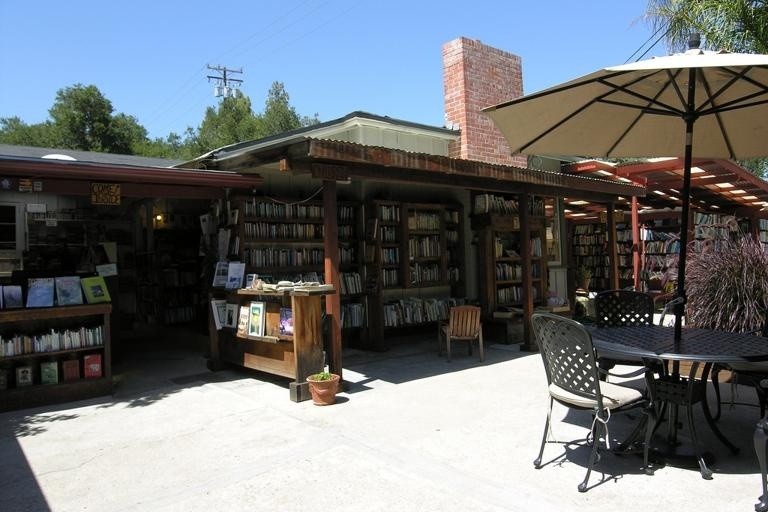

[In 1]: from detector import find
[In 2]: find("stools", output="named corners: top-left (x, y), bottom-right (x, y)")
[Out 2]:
top-left (752, 405), bottom-right (768, 512)
top-left (711, 315), bottom-right (768, 424)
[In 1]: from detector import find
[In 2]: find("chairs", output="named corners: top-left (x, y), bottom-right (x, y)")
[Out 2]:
top-left (594, 289), bottom-right (672, 423)
top-left (436, 304), bottom-right (486, 363)
top-left (530, 311), bottom-right (658, 493)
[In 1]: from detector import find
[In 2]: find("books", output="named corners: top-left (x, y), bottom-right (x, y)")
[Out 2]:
top-left (568, 216), bottom-right (767, 293)
top-left (0, 274), bottom-right (112, 390)
top-left (201, 194), bottom-right (546, 346)
top-left (135, 247), bottom-right (198, 325)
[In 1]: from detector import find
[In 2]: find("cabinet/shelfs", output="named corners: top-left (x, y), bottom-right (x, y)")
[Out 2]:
top-left (0, 301), bottom-right (114, 413)
top-left (695, 210), bottom-right (730, 250)
top-left (569, 214), bottom-right (685, 292)
top-left (205, 283), bottom-right (338, 404)
top-left (366, 198), bottom-right (461, 334)
top-left (242, 199), bottom-right (365, 334)
top-left (469, 192), bottom-right (546, 316)
top-left (24, 211), bottom-right (138, 333)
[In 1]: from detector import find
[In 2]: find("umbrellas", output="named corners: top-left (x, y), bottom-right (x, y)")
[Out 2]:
top-left (478, 32), bottom-right (768, 444)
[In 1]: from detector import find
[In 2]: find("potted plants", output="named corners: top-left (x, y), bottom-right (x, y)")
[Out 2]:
top-left (304, 363), bottom-right (340, 406)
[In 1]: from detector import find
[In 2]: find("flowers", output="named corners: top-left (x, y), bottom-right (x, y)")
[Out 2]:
top-left (643, 218), bottom-right (768, 335)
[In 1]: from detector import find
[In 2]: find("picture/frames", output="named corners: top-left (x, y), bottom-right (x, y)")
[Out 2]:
top-left (248, 300), bottom-right (266, 337)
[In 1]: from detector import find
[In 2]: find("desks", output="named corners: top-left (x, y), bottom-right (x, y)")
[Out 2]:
top-left (583, 324), bottom-right (768, 480)
